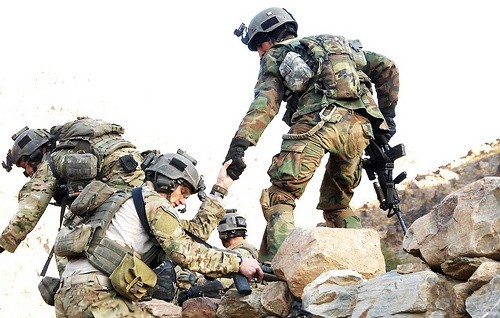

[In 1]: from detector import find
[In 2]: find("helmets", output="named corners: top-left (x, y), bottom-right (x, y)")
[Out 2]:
top-left (2, 126), bottom-right (51, 172)
top-left (234, 7), bottom-right (298, 51)
top-left (217, 209), bottom-right (248, 232)
top-left (144, 150), bottom-right (207, 201)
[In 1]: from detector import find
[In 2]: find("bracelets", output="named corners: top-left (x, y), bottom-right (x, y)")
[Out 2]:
top-left (211, 185), bottom-right (228, 196)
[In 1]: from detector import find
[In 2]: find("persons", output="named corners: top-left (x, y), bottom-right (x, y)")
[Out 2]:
top-left (1, 116), bottom-right (263, 318)
top-left (222, 7), bottom-right (399, 262)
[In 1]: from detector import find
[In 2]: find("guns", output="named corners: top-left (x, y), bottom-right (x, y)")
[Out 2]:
top-left (204, 248), bottom-right (277, 298)
top-left (362, 131), bottom-right (408, 233)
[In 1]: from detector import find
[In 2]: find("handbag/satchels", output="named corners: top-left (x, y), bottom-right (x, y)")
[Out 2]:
top-left (38, 277), bottom-right (60, 306)
top-left (150, 258), bottom-right (177, 301)
top-left (109, 251), bottom-right (157, 302)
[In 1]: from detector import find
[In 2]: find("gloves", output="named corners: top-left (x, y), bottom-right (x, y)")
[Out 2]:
top-left (373, 116), bottom-right (396, 147)
top-left (177, 269), bottom-right (199, 286)
top-left (222, 138), bottom-right (250, 181)
